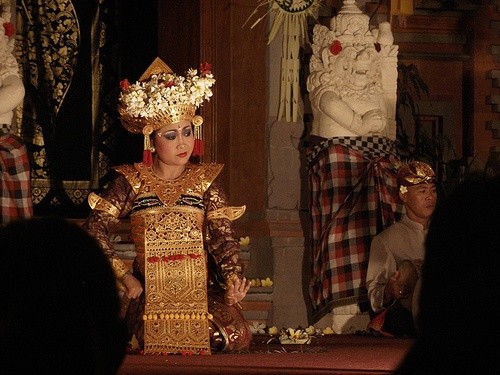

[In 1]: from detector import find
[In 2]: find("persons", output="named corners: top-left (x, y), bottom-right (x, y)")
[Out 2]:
top-left (83, 56), bottom-right (253, 356)
top-left (364, 160), bottom-right (438, 339)
top-left (394, 175), bottom-right (500, 375)
top-left (0, 217), bottom-right (131, 375)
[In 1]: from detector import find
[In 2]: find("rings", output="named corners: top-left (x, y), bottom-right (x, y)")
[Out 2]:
top-left (400, 290), bottom-right (401, 293)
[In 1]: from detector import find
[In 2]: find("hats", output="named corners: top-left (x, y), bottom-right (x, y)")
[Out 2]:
top-left (391, 160), bottom-right (437, 188)
top-left (116, 62), bottom-right (216, 166)
